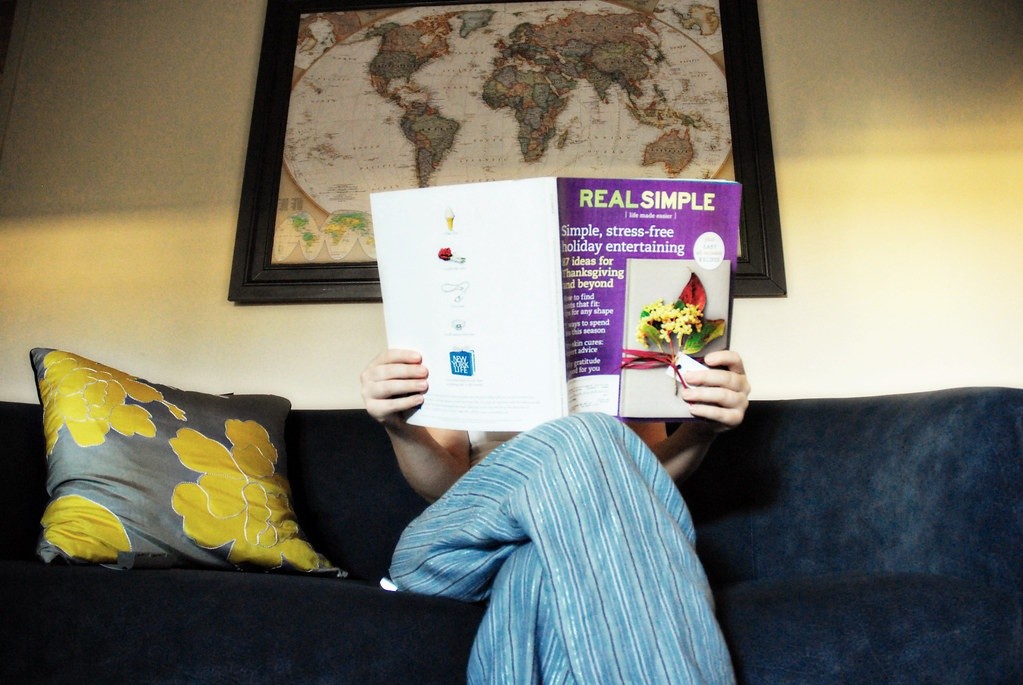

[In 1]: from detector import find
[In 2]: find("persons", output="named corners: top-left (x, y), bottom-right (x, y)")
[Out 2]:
top-left (359, 350), bottom-right (750, 685)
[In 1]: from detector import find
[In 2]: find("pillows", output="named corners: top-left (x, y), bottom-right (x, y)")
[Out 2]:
top-left (28, 347), bottom-right (350, 578)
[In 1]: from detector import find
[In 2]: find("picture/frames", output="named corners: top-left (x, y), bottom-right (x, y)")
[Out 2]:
top-left (226, 0), bottom-right (788, 306)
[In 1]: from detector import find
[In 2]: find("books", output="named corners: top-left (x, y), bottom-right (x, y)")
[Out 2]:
top-left (369, 176), bottom-right (741, 434)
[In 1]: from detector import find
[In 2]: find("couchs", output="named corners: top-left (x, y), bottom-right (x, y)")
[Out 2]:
top-left (0, 387), bottom-right (1023, 685)
